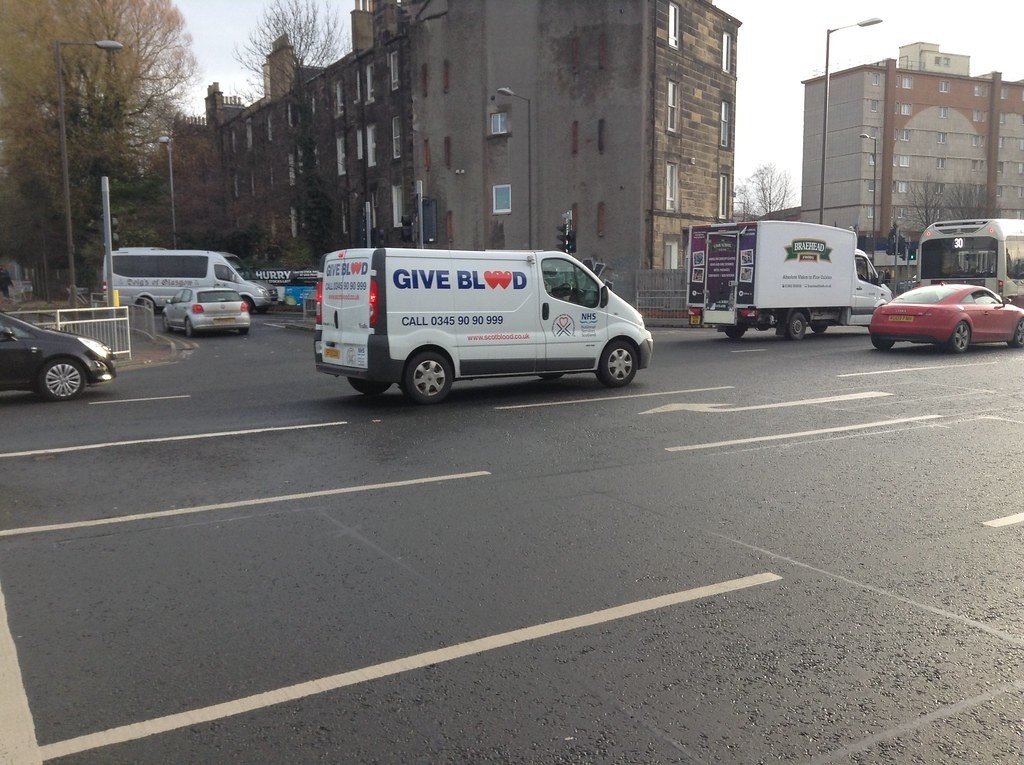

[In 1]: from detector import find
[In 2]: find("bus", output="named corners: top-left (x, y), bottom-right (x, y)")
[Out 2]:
top-left (913, 217), bottom-right (1024, 311)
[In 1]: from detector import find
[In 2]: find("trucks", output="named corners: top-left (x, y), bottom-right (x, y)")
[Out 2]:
top-left (685, 219), bottom-right (893, 341)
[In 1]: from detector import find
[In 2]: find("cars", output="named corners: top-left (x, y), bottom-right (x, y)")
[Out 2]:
top-left (0, 310), bottom-right (120, 402)
top-left (866, 283), bottom-right (1024, 355)
top-left (162, 286), bottom-right (251, 338)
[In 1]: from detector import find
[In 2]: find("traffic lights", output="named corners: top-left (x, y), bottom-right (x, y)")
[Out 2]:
top-left (567, 231), bottom-right (577, 253)
top-left (557, 223), bottom-right (566, 251)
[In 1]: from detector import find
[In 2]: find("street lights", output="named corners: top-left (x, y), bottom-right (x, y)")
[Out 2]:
top-left (157, 135), bottom-right (177, 253)
top-left (55, 38), bottom-right (125, 318)
top-left (497, 85), bottom-right (532, 251)
top-left (819, 18), bottom-right (885, 224)
top-left (857, 132), bottom-right (876, 268)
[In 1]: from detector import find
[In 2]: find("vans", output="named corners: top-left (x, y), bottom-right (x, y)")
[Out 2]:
top-left (102, 247), bottom-right (281, 319)
top-left (314, 245), bottom-right (653, 406)
